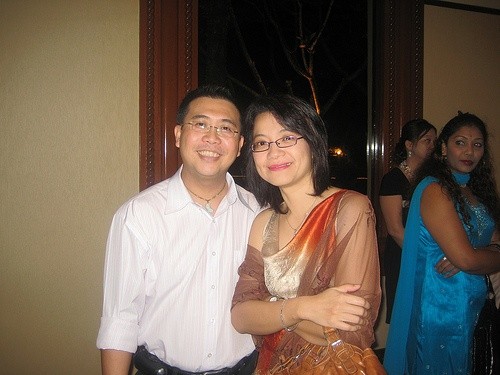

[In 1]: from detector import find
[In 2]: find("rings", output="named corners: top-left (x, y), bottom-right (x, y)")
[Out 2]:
top-left (443, 256), bottom-right (447, 261)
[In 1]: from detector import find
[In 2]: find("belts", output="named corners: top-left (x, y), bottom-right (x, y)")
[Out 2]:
top-left (160, 351), bottom-right (257, 375)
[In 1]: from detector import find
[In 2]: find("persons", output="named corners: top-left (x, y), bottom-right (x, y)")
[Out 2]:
top-left (230, 95), bottom-right (388, 375)
top-left (96, 84), bottom-right (271, 375)
top-left (379, 118), bottom-right (438, 324)
top-left (382, 112), bottom-right (500, 375)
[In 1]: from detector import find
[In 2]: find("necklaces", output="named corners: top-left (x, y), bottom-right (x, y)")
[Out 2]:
top-left (399, 159), bottom-right (412, 176)
top-left (185, 182), bottom-right (226, 213)
top-left (465, 196), bottom-right (489, 236)
top-left (286, 197), bottom-right (317, 234)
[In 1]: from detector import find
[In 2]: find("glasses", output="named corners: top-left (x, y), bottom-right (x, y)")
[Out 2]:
top-left (251, 135), bottom-right (304, 152)
top-left (182, 122), bottom-right (240, 137)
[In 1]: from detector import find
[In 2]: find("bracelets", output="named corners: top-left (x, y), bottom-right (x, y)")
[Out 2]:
top-left (280, 299), bottom-right (299, 331)
